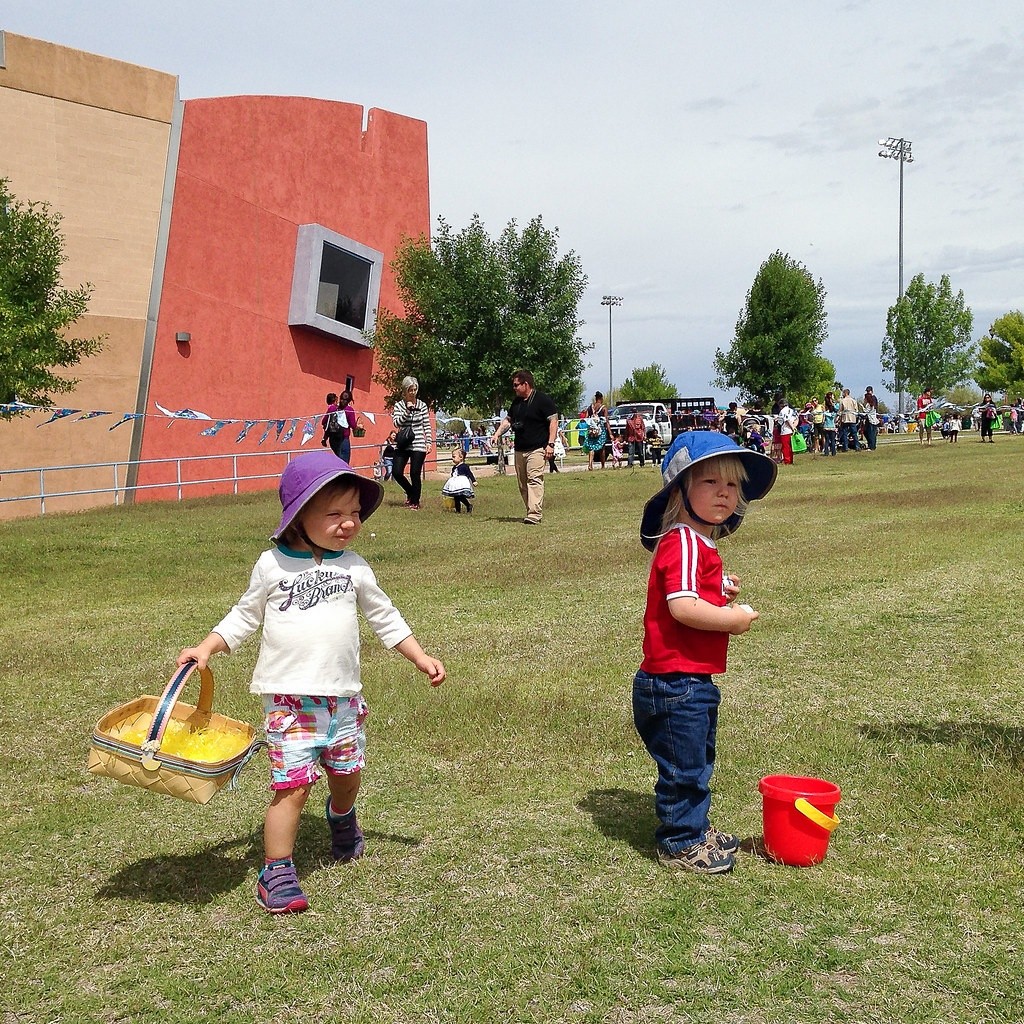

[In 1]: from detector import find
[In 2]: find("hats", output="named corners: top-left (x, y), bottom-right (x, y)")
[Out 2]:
top-left (267, 450), bottom-right (385, 548)
top-left (805, 402), bottom-right (812, 408)
top-left (639, 430), bottom-right (778, 554)
top-left (865, 386), bottom-right (872, 391)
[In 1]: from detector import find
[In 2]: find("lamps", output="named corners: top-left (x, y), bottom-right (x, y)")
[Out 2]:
top-left (176, 332), bottom-right (193, 344)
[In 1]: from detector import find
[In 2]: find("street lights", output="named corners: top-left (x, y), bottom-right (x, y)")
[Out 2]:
top-left (878, 137), bottom-right (915, 433)
top-left (600, 295), bottom-right (623, 407)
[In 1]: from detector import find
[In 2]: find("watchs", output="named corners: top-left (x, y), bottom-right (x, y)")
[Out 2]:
top-left (547, 442), bottom-right (555, 448)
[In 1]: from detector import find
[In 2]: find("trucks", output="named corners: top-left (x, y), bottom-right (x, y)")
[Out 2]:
top-left (606, 397), bottom-right (714, 459)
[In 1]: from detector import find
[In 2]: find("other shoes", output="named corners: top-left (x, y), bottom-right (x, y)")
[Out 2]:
top-left (842, 449), bottom-right (847, 452)
top-left (856, 448), bottom-right (861, 452)
top-left (866, 448), bottom-right (874, 451)
top-left (467, 504), bottom-right (474, 513)
top-left (523, 517), bottom-right (541, 524)
top-left (409, 503), bottom-right (419, 512)
top-left (981, 440), bottom-right (985, 442)
top-left (405, 497), bottom-right (412, 508)
top-left (989, 440), bottom-right (995, 443)
top-left (760, 441), bottom-right (770, 448)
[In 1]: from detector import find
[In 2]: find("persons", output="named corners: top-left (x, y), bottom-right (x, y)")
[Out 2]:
top-left (979, 394), bottom-right (997, 443)
top-left (391, 374), bottom-right (432, 512)
top-left (373, 430), bottom-right (397, 483)
top-left (441, 448), bottom-right (479, 514)
top-left (437, 413), bottom-right (571, 476)
top-left (321, 390), bottom-right (360, 466)
top-left (490, 369), bottom-right (559, 524)
top-left (877, 407), bottom-right (972, 443)
top-left (917, 388), bottom-right (934, 446)
top-left (575, 387), bottom-right (868, 472)
top-left (863, 385), bottom-right (874, 449)
top-left (1009, 404), bottom-right (1020, 436)
top-left (632, 429), bottom-right (760, 874)
top-left (859, 393), bottom-right (877, 452)
top-left (175, 451), bottom-right (447, 914)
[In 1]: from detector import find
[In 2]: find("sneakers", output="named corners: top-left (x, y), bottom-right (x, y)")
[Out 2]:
top-left (326, 794), bottom-right (365, 863)
top-left (656, 843), bottom-right (736, 874)
top-left (702, 825), bottom-right (739, 854)
top-left (254, 859), bottom-right (308, 913)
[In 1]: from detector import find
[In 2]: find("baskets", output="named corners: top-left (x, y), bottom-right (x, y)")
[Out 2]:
top-left (352, 416), bottom-right (366, 437)
top-left (443, 495), bottom-right (456, 508)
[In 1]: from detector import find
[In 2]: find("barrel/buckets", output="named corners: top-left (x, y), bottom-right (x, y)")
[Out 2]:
top-left (758, 773), bottom-right (842, 867)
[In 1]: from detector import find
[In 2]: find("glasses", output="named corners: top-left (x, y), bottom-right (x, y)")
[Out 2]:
top-left (513, 382), bottom-right (521, 387)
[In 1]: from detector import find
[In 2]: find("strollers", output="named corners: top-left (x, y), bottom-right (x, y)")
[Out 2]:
top-left (739, 417), bottom-right (765, 454)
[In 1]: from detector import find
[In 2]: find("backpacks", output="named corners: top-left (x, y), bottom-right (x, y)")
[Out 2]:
top-left (588, 404), bottom-right (605, 438)
top-left (324, 408), bottom-right (346, 438)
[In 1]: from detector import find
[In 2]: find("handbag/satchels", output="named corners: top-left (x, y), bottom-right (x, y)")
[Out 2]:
top-left (790, 429), bottom-right (808, 454)
top-left (395, 427), bottom-right (415, 450)
top-left (990, 414), bottom-right (1001, 430)
top-left (87, 660), bottom-right (257, 805)
top-left (986, 408), bottom-right (996, 419)
top-left (925, 411), bottom-right (942, 427)
top-left (383, 440), bottom-right (395, 457)
